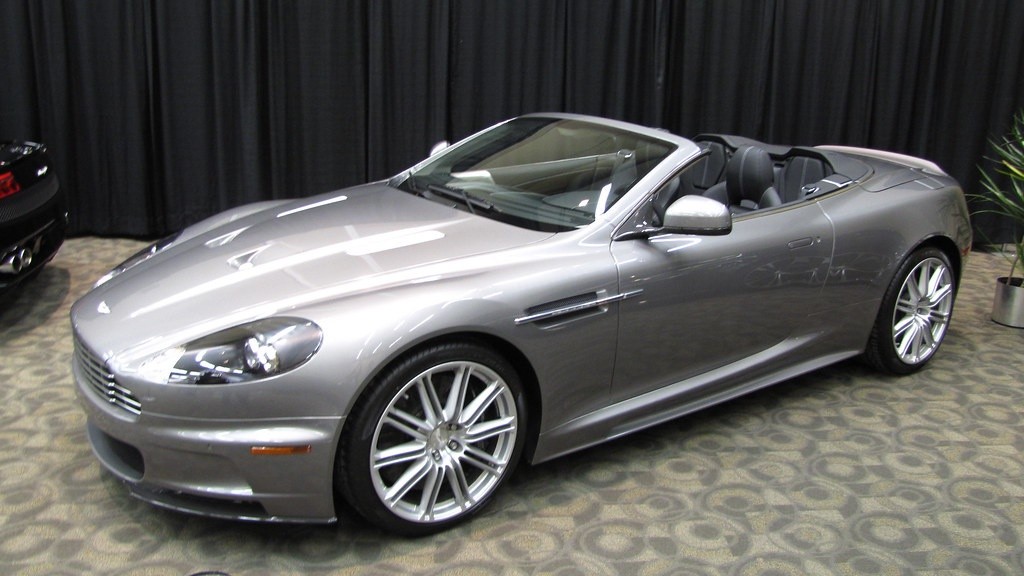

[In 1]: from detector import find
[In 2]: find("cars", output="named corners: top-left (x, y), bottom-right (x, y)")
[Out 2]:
top-left (0, 140), bottom-right (66, 293)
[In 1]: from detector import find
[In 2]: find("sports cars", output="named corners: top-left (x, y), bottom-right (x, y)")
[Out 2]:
top-left (71, 113), bottom-right (970, 540)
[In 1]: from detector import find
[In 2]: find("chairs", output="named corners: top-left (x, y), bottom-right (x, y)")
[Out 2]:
top-left (703, 144), bottom-right (781, 214)
top-left (611, 127), bottom-right (682, 227)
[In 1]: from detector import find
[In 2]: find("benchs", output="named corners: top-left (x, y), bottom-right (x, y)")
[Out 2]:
top-left (681, 141), bottom-right (834, 209)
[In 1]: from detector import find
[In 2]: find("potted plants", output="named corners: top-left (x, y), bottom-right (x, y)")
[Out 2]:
top-left (964, 111), bottom-right (1024, 330)
